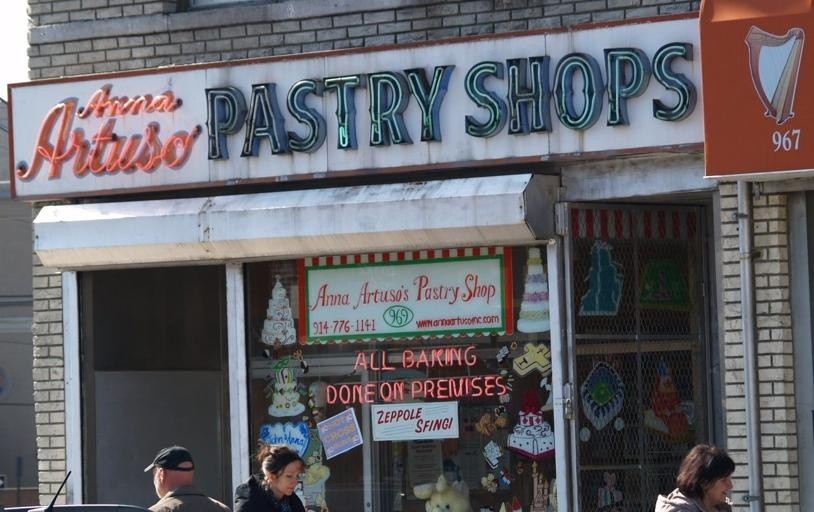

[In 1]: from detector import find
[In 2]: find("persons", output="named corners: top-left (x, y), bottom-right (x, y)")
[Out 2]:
top-left (232, 439), bottom-right (306, 512)
top-left (654, 443), bottom-right (736, 512)
top-left (144, 445), bottom-right (231, 512)
top-left (443, 458), bottom-right (470, 497)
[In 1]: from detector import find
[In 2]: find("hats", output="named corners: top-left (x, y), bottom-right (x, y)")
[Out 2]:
top-left (144, 446), bottom-right (196, 473)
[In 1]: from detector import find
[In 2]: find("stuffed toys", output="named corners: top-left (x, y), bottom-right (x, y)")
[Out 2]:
top-left (413, 473), bottom-right (474, 512)
